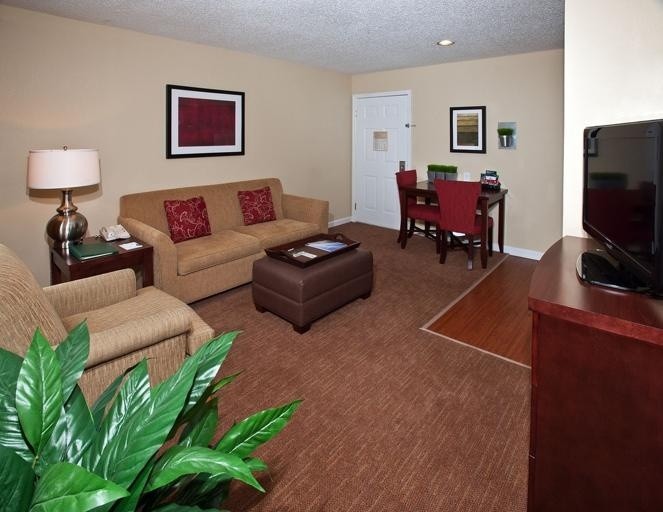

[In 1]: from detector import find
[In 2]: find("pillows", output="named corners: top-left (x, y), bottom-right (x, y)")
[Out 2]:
top-left (163, 195), bottom-right (210, 244)
top-left (236, 186), bottom-right (274, 226)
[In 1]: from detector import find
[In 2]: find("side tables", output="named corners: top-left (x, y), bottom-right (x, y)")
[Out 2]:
top-left (51, 238), bottom-right (152, 289)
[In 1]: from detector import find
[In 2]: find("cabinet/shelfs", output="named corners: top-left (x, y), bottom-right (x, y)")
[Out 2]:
top-left (526, 235), bottom-right (661, 512)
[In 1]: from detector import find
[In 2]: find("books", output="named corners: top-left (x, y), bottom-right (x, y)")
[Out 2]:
top-left (305, 239), bottom-right (349, 253)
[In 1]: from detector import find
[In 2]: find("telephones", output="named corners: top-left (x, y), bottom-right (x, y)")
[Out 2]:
top-left (99, 224), bottom-right (131, 242)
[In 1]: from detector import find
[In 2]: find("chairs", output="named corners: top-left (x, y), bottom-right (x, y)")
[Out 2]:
top-left (395, 170), bottom-right (493, 271)
top-left (0, 241), bottom-right (213, 411)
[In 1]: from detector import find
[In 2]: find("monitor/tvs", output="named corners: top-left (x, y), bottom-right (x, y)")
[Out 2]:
top-left (576, 119), bottom-right (662, 291)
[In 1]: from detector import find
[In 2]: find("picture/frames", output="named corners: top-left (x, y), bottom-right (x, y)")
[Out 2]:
top-left (164, 83), bottom-right (244, 158)
top-left (450, 106), bottom-right (486, 153)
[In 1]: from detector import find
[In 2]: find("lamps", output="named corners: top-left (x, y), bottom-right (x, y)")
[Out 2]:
top-left (26, 147), bottom-right (101, 250)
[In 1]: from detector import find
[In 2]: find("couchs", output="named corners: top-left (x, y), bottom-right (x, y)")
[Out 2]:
top-left (116, 178), bottom-right (329, 304)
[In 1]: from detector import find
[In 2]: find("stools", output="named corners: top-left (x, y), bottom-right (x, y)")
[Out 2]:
top-left (251, 244), bottom-right (373, 334)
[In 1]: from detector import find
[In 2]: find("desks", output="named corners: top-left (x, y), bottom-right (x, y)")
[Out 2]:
top-left (400, 179), bottom-right (506, 271)
